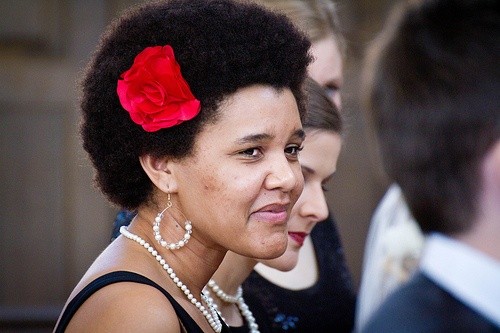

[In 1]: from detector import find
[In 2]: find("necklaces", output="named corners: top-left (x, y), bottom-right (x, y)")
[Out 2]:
top-left (203, 279), bottom-right (262, 333)
top-left (120, 225), bottom-right (222, 333)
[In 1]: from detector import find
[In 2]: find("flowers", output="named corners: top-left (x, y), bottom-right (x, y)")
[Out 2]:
top-left (117, 45), bottom-right (201, 132)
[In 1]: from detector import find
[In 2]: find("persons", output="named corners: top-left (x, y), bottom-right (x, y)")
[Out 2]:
top-left (46, 0), bottom-right (311, 333)
top-left (239, 0), bottom-right (358, 333)
top-left (359, 0), bottom-right (500, 333)
top-left (199, 73), bottom-right (343, 333)
